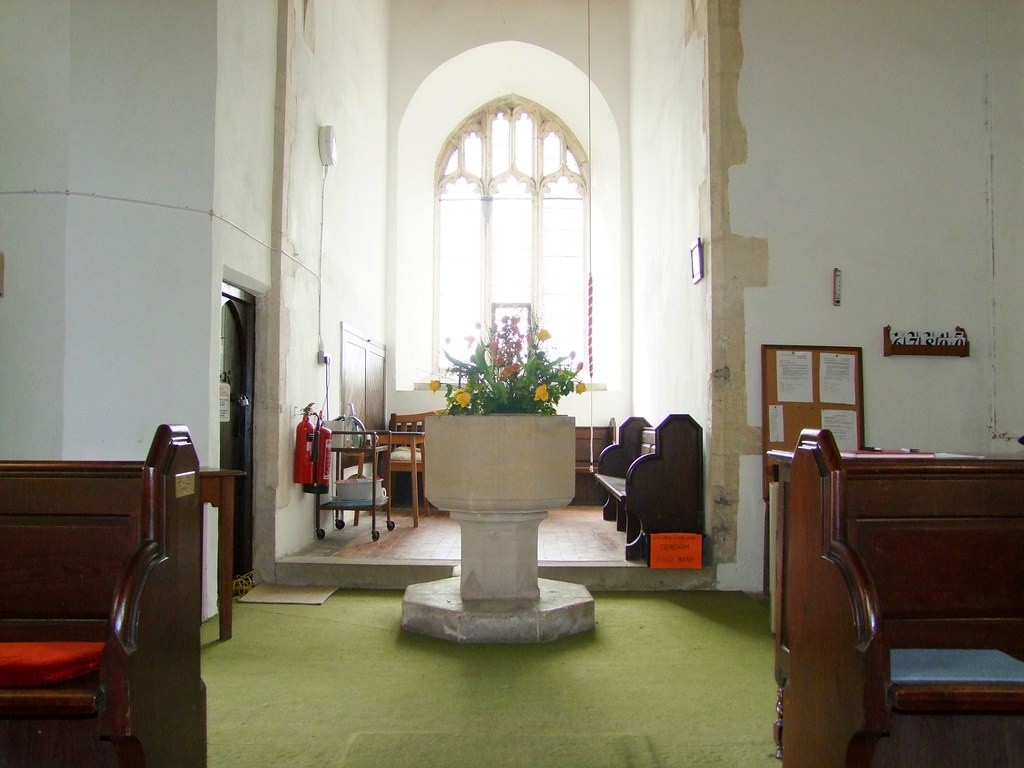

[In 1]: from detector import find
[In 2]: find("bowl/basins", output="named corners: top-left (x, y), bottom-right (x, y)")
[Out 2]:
top-left (335, 478), bottom-right (383, 499)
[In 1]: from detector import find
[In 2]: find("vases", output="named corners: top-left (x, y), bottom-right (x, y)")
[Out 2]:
top-left (402, 413), bottom-right (594, 644)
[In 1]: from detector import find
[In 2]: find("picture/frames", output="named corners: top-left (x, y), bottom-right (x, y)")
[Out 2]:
top-left (691, 238), bottom-right (703, 284)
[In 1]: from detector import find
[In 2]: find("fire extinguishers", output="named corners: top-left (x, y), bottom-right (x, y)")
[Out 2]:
top-left (293, 403), bottom-right (320, 484)
top-left (303, 410), bottom-right (332, 494)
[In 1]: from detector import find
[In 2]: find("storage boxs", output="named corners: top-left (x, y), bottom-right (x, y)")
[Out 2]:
top-left (335, 479), bottom-right (384, 501)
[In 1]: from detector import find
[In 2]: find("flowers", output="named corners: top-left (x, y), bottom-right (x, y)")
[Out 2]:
top-left (429, 313), bottom-right (587, 416)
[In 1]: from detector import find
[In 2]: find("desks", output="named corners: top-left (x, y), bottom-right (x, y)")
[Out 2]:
top-left (377, 432), bottom-right (430, 527)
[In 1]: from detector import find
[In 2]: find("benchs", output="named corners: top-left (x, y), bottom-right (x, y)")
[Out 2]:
top-left (595, 414), bottom-right (702, 561)
top-left (0, 426), bottom-right (209, 768)
top-left (575, 418), bottom-right (616, 505)
top-left (778, 428), bottom-right (1024, 768)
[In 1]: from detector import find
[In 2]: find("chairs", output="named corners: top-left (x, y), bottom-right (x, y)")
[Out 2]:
top-left (389, 412), bottom-right (433, 510)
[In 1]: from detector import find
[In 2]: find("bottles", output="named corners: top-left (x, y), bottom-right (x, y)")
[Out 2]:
top-left (344, 403), bottom-right (359, 449)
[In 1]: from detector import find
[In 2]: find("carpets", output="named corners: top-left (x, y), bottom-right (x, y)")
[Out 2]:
top-left (238, 583), bottom-right (340, 604)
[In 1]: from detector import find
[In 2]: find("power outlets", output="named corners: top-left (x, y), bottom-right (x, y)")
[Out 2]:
top-left (319, 351), bottom-right (330, 364)
